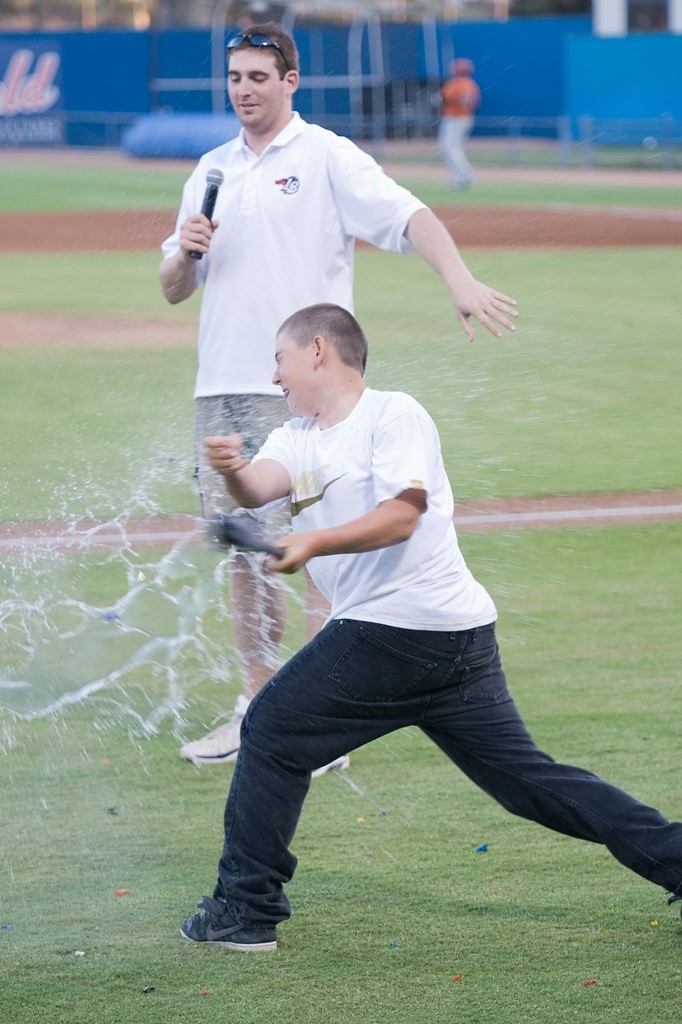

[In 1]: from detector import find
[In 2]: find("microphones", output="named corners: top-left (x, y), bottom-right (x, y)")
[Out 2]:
top-left (189, 168), bottom-right (224, 259)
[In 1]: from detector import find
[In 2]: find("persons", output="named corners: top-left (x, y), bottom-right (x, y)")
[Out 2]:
top-left (431, 60), bottom-right (477, 188)
top-left (159, 26), bottom-right (519, 775)
top-left (181, 303), bottom-right (681, 950)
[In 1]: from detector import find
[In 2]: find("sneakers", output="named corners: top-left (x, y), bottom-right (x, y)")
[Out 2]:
top-left (179, 895), bottom-right (277, 952)
top-left (180, 694), bottom-right (251, 763)
top-left (312, 753), bottom-right (352, 778)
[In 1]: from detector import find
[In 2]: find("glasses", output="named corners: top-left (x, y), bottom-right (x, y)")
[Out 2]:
top-left (226, 32), bottom-right (290, 70)
